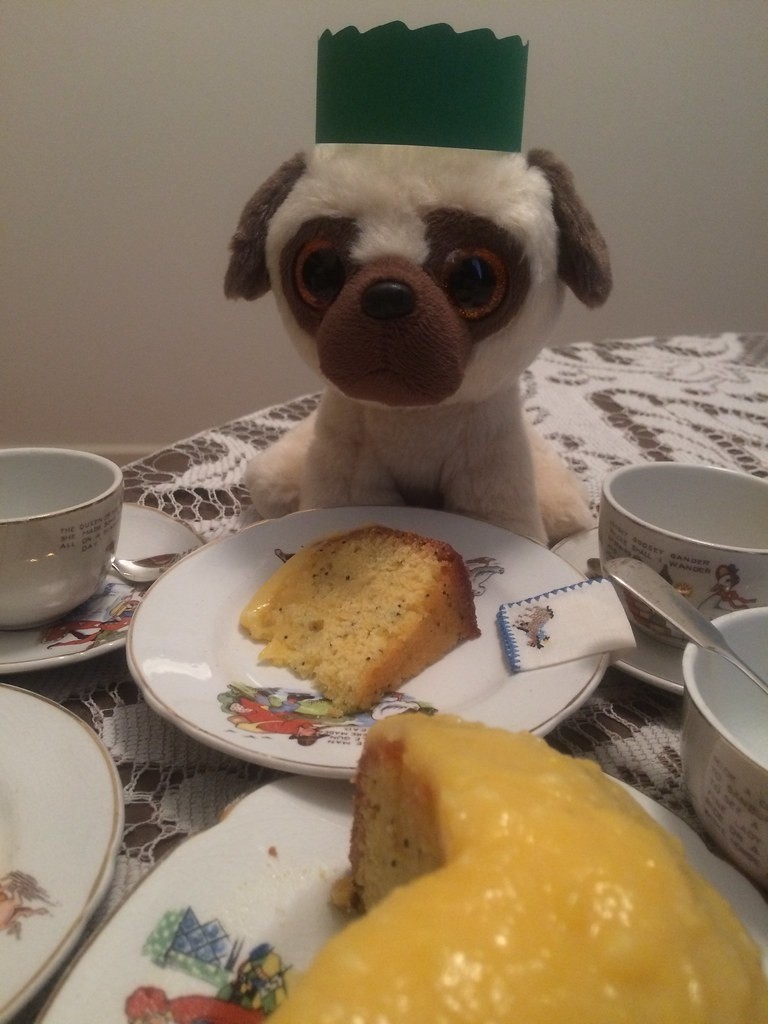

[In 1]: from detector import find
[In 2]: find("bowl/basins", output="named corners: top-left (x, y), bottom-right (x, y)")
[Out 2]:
top-left (0, 447), bottom-right (125, 633)
top-left (681, 606), bottom-right (767, 888)
top-left (597, 462), bottom-right (767, 647)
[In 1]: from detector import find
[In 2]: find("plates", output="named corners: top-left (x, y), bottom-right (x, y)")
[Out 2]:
top-left (1, 684), bottom-right (124, 1023)
top-left (124, 506), bottom-right (609, 780)
top-left (0, 504), bottom-right (206, 674)
top-left (548, 526), bottom-right (685, 697)
top-left (40, 779), bottom-right (767, 1024)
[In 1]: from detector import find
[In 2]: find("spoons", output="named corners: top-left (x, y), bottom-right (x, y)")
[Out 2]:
top-left (109, 552), bottom-right (183, 582)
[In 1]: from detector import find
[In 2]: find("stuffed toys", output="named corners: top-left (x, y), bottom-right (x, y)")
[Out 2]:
top-left (224, 143), bottom-right (614, 548)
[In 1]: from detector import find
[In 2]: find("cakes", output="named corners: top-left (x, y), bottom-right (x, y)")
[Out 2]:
top-left (250, 709), bottom-right (767, 1024)
top-left (237, 523), bottom-right (481, 717)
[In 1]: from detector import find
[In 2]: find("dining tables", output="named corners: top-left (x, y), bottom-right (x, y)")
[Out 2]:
top-left (0, 328), bottom-right (768, 1024)
